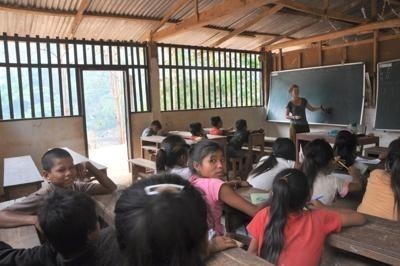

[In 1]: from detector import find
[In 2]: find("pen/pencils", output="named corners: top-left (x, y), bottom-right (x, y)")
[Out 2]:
top-left (315, 195), bottom-right (324, 200)
top-left (334, 156), bottom-right (350, 171)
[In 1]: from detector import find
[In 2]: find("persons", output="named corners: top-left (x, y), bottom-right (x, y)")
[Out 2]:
top-left (114, 171), bottom-right (215, 265)
top-left (153, 135), bottom-right (192, 183)
top-left (207, 116), bottom-right (233, 136)
top-left (0, 148), bottom-right (117, 229)
top-left (284, 84), bottom-right (328, 161)
top-left (226, 118), bottom-right (264, 181)
top-left (183, 122), bottom-right (207, 142)
top-left (246, 168), bottom-right (367, 266)
top-left (186, 140), bottom-right (270, 237)
top-left (141, 120), bottom-right (168, 161)
top-left (0, 187), bottom-right (121, 265)
top-left (245, 130), bottom-right (400, 222)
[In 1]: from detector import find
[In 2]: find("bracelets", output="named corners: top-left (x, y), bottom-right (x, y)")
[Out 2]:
top-left (294, 116), bottom-right (296, 120)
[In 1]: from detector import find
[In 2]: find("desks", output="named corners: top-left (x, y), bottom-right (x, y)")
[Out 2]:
top-left (0, 125), bottom-right (400, 266)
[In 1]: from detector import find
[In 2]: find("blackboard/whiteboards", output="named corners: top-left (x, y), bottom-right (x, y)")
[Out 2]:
top-left (264, 61), bottom-right (366, 126)
top-left (373, 59), bottom-right (400, 132)
